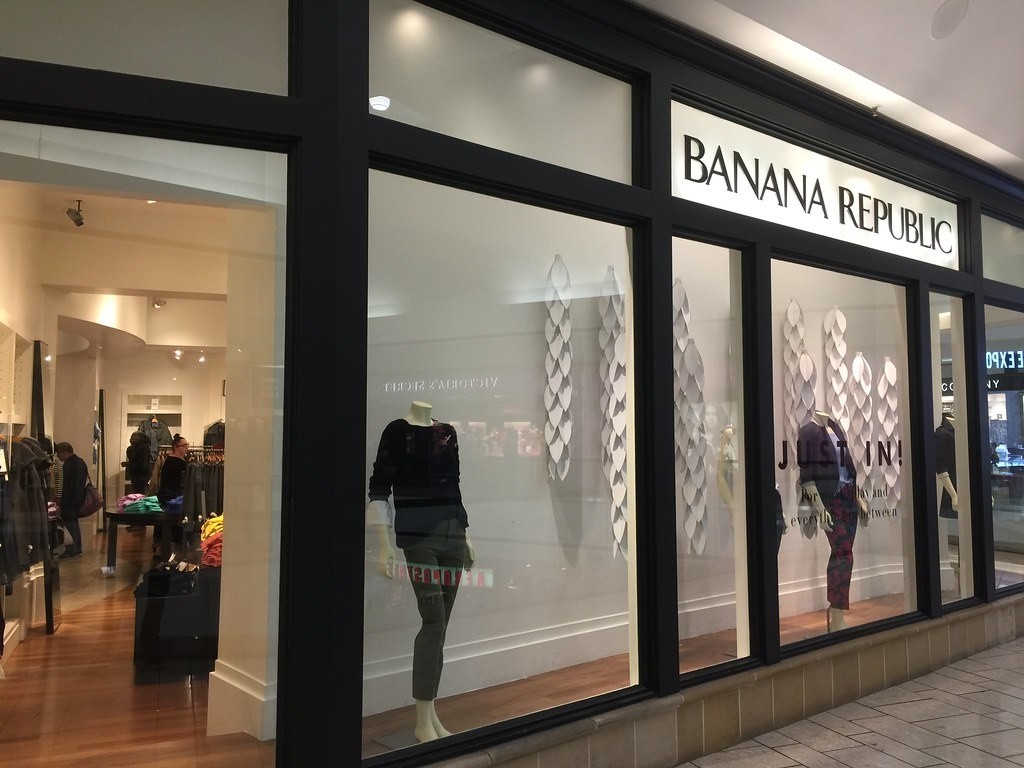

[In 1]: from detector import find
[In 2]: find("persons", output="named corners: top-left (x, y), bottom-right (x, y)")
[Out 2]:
top-left (152, 433), bottom-right (187, 560)
top-left (56, 442), bottom-right (88, 558)
top-left (121, 431), bottom-right (152, 532)
top-left (368, 401), bottom-right (474, 743)
top-left (991, 440), bottom-right (1009, 460)
top-left (934, 414), bottom-right (998, 599)
top-left (796, 409), bottom-right (870, 631)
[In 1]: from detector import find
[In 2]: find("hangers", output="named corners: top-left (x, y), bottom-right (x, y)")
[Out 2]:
top-left (219, 418), bottom-right (224, 426)
top-left (0, 434), bottom-right (22, 443)
top-left (152, 414), bottom-right (158, 423)
top-left (159, 442), bottom-right (224, 465)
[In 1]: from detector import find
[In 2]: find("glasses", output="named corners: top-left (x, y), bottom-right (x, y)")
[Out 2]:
top-left (176, 443), bottom-right (189, 447)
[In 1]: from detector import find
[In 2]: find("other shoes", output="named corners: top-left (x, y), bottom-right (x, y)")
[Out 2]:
top-left (125, 526), bottom-right (147, 531)
top-left (58, 552), bottom-right (84, 559)
top-left (153, 554), bottom-right (162, 559)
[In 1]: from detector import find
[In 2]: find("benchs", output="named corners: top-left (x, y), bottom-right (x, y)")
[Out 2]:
top-left (133, 566), bottom-right (221, 665)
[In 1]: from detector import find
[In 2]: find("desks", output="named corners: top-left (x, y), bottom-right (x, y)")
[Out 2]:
top-left (103, 504), bottom-right (179, 572)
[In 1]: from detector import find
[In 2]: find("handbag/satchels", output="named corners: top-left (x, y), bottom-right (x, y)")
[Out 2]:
top-left (78, 483), bottom-right (104, 518)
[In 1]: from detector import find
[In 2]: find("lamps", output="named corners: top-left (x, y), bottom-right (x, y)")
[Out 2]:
top-left (151, 300), bottom-right (166, 309)
top-left (42, 352), bottom-right (52, 362)
